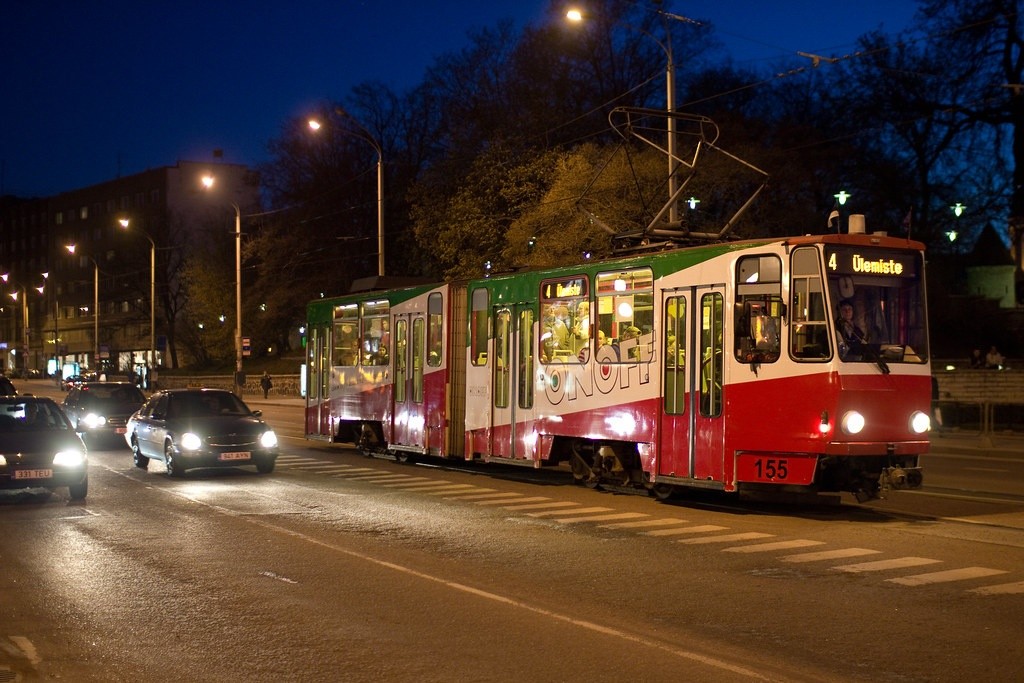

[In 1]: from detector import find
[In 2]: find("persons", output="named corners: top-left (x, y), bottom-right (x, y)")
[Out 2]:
top-left (20, 403), bottom-right (47, 426)
top-left (715, 329), bottom-right (722, 367)
top-left (836, 303), bottom-right (869, 356)
top-left (667, 336), bottom-right (685, 368)
top-left (208, 398), bottom-right (230, 415)
top-left (931, 367), bottom-right (943, 438)
top-left (117, 388), bottom-right (132, 401)
top-left (970, 346), bottom-right (1002, 370)
top-left (497, 301), bottom-right (641, 363)
top-left (339, 321), bottom-right (441, 366)
top-left (261, 371), bottom-right (273, 399)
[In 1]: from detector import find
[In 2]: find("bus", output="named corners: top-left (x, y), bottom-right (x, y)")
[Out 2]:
top-left (304, 171), bottom-right (932, 509)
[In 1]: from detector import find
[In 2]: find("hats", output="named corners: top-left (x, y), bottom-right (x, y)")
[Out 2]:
top-left (838, 298), bottom-right (856, 310)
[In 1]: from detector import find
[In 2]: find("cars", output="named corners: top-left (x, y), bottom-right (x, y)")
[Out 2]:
top-left (60, 382), bottom-right (147, 445)
top-left (0, 375), bottom-right (89, 500)
top-left (123, 386), bottom-right (281, 477)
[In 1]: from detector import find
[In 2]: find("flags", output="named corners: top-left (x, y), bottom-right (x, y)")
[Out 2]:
top-left (828, 201), bottom-right (839, 227)
top-left (903, 211), bottom-right (912, 233)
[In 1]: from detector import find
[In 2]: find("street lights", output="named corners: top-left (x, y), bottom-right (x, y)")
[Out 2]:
top-left (0, 272), bottom-right (28, 380)
top-left (942, 200), bottom-right (967, 269)
top-left (833, 191), bottom-right (851, 233)
top-left (564, 7), bottom-right (681, 225)
top-left (63, 244), bottom-right (99, 368)
top-left (199, 172), bottom-right (242, 400)
top-left (117, 218), bottom-right (157, 393)
top-left (306, 108), bottom-right (385, 276)
